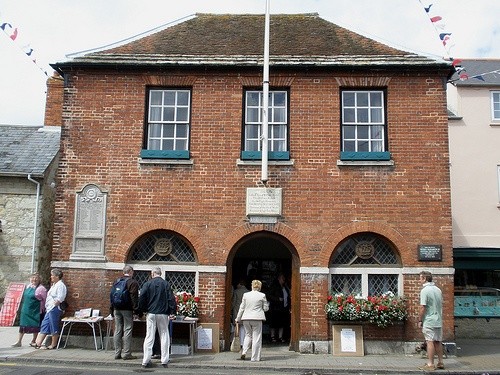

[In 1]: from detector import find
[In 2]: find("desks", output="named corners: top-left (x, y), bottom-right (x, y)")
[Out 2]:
top-left (56, 316), bottom-right (104, 350)
top-left (103, 314), bottom-right (199, 356)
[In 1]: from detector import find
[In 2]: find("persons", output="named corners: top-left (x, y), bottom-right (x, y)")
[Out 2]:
top-left (230, 280), bottom-right (250, 350)
top-left (417, 271), bottom-right (444, 370)
top-left (11, 271), bottom-right (47, 347)
top-left (110, 266), bottom-right (144, 360)
top-left (138, 266), bottom-right (177, 366)
top-left (235, 280), bottom-right (270, 362)
top-left (266, 273), bottom-right (291, 343)
top-left (30, 268), bottom-right (68, 349)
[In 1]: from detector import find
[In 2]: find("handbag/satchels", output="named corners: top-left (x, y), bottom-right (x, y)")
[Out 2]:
top-left (60, 301), bottom-right (68, 310)
top-left (230, 323), bottom-right (240, 352)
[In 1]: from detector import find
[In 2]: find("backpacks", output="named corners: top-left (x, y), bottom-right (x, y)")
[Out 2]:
top-left (110, 278), bottom-right (132, 310)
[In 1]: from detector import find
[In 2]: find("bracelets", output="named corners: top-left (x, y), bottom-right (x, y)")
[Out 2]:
top-left (419, 321), bottom-right (422, 322)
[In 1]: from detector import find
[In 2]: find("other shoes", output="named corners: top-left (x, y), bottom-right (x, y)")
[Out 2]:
top-left (162, 360), bottom-right (170, 364)
top-left (241, 354), bottom-right (246, 360)
top-left (269, 336), bottom-right (277, 344)
top-left (123, 354), bottom-right (137, 360)
top-left (278, 336), bottom-right (285, 344)
top-left (142, 360), bottom-right (153, 366)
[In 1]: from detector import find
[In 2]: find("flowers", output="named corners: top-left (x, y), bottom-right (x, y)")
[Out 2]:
top-left (172, 288), bottom-right (200, 316)
top-left (326, 290), bottom-right (409, 321)
